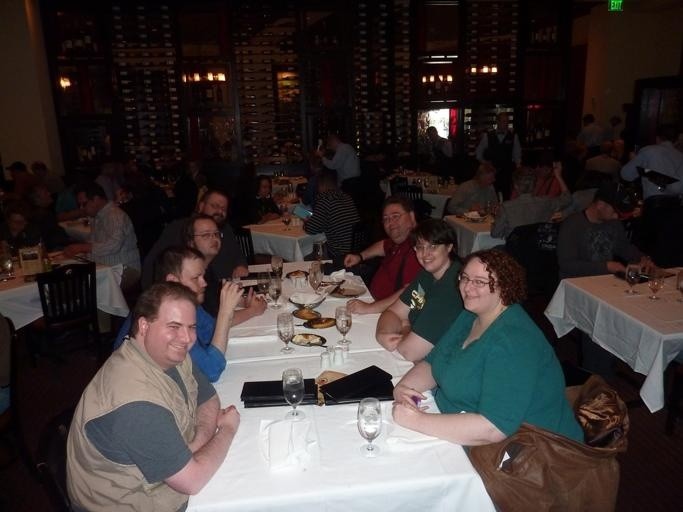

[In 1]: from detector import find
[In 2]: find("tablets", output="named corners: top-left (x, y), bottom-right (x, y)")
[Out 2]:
top-left (292, 205), bottom-right (314, 220)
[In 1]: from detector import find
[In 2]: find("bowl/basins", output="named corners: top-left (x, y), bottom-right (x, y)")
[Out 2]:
top-left (463, 210), bottom-right (489, 222)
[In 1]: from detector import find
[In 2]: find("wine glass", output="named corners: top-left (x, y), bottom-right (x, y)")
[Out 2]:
top-left (255, 268), bottom-right (271, 306)
top-left (281, 214), bottom-right (292, 232)
top-left (1, 245), bottom-right (18, 280)
top-left (626, 263), bottom-right (683, 304)
top-left (268, 254), bottom-right (385, 459)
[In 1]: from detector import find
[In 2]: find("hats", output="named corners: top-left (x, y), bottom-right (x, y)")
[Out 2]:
top-left (593, 183), bottom-right (629, 212)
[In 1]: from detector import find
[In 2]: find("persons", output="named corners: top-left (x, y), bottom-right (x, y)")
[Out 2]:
top-left (64, 280), bottom-right (241, 512)
top-left (391, 248), bottom-right (584, 458)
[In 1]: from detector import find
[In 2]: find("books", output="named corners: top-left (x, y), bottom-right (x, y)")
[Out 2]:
top-left (240, 366), bottom-right (396, 408)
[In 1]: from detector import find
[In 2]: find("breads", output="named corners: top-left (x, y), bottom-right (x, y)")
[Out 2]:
top-left (302, 317), bottom-right (336, 329)
top-left (293, 308), bottom-right (321, 321)
top-left (291, 270), bottom-right (305, 278)
top-left (292, 334), bottom-right (321, 345)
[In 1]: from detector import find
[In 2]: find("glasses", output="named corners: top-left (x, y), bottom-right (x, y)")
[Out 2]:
top-left (456, 273), bottom-right (499, 288)
top-left (189, 231), bottom-right (224, 240)
top-left (382, 212), bottom-right (408, 222)
top-left (412, 242), bottom-right (447, 253)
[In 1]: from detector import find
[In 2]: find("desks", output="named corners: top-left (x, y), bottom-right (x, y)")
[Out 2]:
top-left (2, 153), bottom-right (683, 511)
